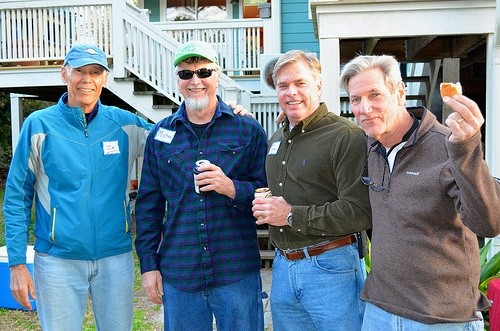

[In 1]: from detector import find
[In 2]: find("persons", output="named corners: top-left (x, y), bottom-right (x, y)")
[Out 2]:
top-left (337, 55), bottom-right (500, 331)
top-left (4, 44), bottom-right (257, 331)
top-left (249, 49), bottom-right (372, 331)
top-left (137, 42), bottom-right (269, 331)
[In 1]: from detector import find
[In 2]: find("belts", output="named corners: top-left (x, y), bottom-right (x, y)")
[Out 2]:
top-left (280, 233), bottom-right (356, 261)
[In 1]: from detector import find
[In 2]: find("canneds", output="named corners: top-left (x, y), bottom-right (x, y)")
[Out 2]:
top-left (192, 160), bottom-right (212, 195)
top-left (253, 188), bottom-right (272, 220)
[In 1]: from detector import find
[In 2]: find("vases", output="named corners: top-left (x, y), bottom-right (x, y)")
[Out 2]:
top-left (14, 39), bottom-right (41, 65)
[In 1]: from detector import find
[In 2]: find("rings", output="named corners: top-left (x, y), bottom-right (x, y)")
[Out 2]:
top-left (459, 118), bottom-right (465, 124)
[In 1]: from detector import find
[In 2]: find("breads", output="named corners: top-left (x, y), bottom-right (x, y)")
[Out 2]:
top-left (440, 82), bottom-right (462, 98)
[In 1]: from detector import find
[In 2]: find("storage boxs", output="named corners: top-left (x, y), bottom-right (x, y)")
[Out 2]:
top-left (258, 2), bottom-right (271, 18)
top-left (0, 245), bottom-right (37, 311)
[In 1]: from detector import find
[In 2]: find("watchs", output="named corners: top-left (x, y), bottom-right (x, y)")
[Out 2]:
top-left (286, 208), bottom-right (292, 226)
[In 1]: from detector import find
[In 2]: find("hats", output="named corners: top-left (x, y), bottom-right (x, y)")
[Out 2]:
top-left (64, 44), bottom-right (110, 72)
top-left (173, 40), bottom-right (217, 67)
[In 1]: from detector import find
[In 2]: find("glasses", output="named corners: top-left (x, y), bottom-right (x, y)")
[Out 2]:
top-left (360, 145), bottom-right (393, 191)
top-left (178, 67), bottom-right (218, 80)
